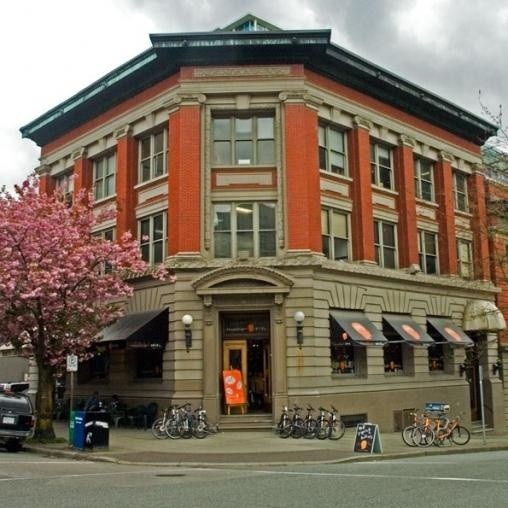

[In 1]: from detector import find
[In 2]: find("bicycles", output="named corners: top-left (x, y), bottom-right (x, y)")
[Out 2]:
top-left (275, 401), bottom-right (344, 440)
top-left (149, 401), bottom-right (211, 439)
top-left (401, 406), bottom-right (471, 447)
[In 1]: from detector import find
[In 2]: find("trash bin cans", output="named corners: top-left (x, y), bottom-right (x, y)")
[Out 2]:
top-left (68, 411), bottom-right (110, 453)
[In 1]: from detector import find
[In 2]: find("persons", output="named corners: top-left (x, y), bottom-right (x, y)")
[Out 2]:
top-left (78, 391), bottom-right (120, 412)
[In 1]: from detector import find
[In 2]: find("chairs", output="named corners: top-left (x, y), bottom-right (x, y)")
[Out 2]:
top-left (111, 402), bottom-right (157, 431)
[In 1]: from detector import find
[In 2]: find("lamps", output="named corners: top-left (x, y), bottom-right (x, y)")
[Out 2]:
top-left (294, 311), bottom-right (305, 349)
top-left (182, 314), bottom-right (193, 353)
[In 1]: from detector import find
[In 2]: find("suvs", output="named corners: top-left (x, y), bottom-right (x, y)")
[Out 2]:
top-left (0, 389), bottom-right (35, 451)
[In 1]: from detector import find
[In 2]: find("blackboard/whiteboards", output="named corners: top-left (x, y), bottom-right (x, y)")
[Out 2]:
top-left (353, 423), bottom-right (377, 455)
